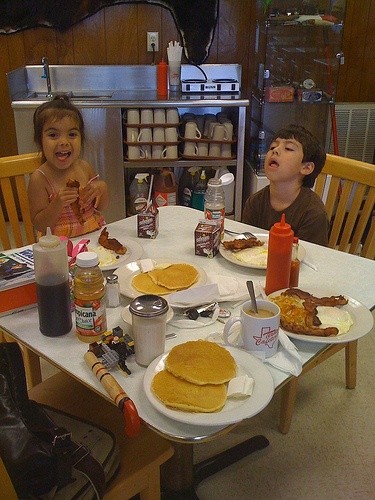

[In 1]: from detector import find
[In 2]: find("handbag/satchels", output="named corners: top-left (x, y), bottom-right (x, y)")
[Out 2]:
top-left (0, 342), bottom-right (105, 500)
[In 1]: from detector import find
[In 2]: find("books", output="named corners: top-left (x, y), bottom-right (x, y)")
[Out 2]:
top-left (0, 243), bottom-right (71, 292)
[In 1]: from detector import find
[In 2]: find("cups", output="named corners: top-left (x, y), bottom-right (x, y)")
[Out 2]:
top-left (168, 60), bottom-right (182, 92)
top-left (222, 300), bottom-right (281, 359)
top-left (124, 109), bottom-right (234, 161)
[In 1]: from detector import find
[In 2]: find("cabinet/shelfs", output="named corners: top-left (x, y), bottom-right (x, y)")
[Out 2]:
top-left (14, 106), bottom-right (246, 229)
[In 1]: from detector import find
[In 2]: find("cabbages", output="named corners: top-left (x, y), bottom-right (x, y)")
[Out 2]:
top-left (87, 247), bottom-right (118, 268)
top-left (233, 245), bottom-right (268, 266)
top-left (316, 304), bottom-right (353, 335)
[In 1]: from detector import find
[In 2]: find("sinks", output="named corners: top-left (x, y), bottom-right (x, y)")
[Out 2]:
top-left (68, 90), bottom-right (114, 100)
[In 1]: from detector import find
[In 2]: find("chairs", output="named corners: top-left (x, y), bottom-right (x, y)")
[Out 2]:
top-left (0, 152), bottom-right (49, 391)
top-left (0, 371), bottom-right (175, 500)
top-left (280, 153), bottom-right (375, 435)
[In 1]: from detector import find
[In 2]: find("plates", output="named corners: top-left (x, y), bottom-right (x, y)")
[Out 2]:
top-left (110, 258), bottom-right (208, 300)
top-left (219, 234), bottom-right (305, 267)
top-left (265, 286), bottom-right (375, 343)
top-left (142, 341), bottom-right (275, 426)
top-left (80, 237), bottom-right (142, 271)
top-left (120, 303), bottom-right (174, 327)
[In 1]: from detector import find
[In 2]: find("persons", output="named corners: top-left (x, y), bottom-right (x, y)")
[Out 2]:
top-left (240, 124), bottom-right (329, 248)
top-left (28, 94), bottom-right (110, 243)
top-left (0, 263), bottom-right (27, 275)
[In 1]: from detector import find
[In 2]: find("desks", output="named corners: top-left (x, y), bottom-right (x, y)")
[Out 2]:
top-left (0, 206), bottom-right (375, 500)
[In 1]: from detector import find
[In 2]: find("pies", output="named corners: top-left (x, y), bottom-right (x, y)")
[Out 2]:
top-left (132, 272), bottom-right (179, 296)
top-left (166, 339), bottom-right (237, 385)
top-left (152, 370), bottom-right (226, 411)
top-left (147, 263), bottom-right (199, 290)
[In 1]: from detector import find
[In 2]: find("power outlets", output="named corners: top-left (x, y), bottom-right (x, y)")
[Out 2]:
top-left (147, 32), bottom-right (159, 52)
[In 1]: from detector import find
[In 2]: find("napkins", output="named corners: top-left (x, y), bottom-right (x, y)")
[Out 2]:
top-left (263, 327), bottom-right (303, 377)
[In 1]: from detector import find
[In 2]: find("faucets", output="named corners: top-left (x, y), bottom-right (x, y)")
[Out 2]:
top-left (40, 56), bottom-right (54, 98)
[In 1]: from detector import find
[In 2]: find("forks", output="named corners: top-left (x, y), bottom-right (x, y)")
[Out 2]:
top-left (223, 228), bottom-right (257, 239)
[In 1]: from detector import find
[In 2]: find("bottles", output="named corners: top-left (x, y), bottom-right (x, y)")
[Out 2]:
top-left (128, 294), bottom-right (169, 367)
top-left (128, 167), bottom-right (231, 218)
top-left (264, 213), bottom-right (294, 296)
top-left (156, 58), bottom-right (168, 96)
top-left (105, 275), bottom-right (120, 308)
top-left (32, 227), bottom-right (74, 336)
top-left (202, 173), bottom-right (235, 240)
top-left (71, 252), bottom-right (108, 343)
top-left (290, 236), bottom-right (300, 288)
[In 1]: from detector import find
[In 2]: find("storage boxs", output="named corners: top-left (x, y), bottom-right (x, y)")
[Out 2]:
top-left (247, 0), bottom-right (346, 198)
